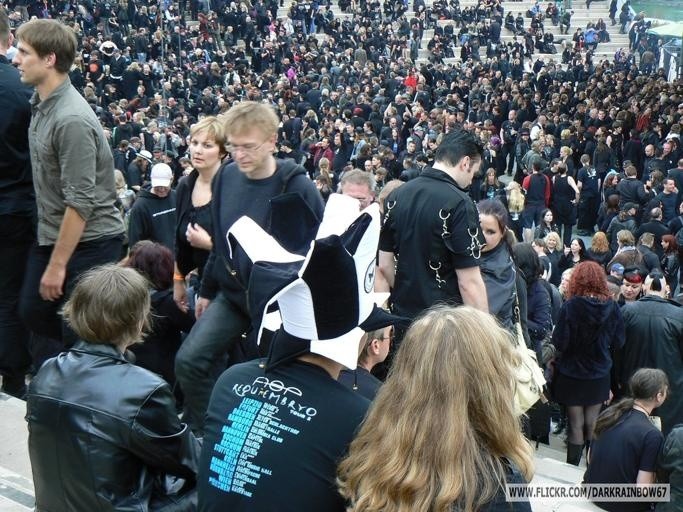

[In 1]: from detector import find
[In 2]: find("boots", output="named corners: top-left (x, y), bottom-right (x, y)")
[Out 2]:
top-left (566, 441), bottom-right (585, 466)
top-left (585, 439), bottom-right (590, 471)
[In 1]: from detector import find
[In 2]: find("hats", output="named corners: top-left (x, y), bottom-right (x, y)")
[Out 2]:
top-left (150, 162), bottom-right (174, 188)
top-left (135, 149), bottom-right (153, 164)
top-left (611, 262), bottom-right (625, 275)
top-left (225, 192), bottom-right (411, 398)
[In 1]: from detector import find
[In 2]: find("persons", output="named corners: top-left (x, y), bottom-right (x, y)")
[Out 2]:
top-left (661, 235), bottom-right (679, 299)
top-left (652, 178), bottom-right (680, 218)
top-left (559, 269), bottom-right (574, 295)
top-left (173, 119), bottom-right (229, 326)
top-left (610, 262), bottom-right (625, 278)
top-left (648, 169), bottom-right (665, 189)
top-left (638, 232), bottom-right (661, 273)
top-left (577, 154), bottom-right (601, 236)
top-left (0, 7), bottom-right (36, 398)
top-left (511, 243), bottom-right (550, 446)
top-left (640, 206), bottom-right (669, 261)
top-left (653, 425), bottom-right (683, 512)
top-left (535, 208), bottom-right (559, 242)
top-left (539, 257), bottom-right (562, 322)
top-left (666, 202), bottom-right (683, 293)
top-left (306, 170), bottom-right (421, 211)
top-left (337, 304), bottom-right (414, 401)
top-left (544, 260), bottom-right (625, 466)
top-left (544, 231), bottom-right (567, 287)
top-left (601, 207), bottom-right (616, 232)
top-left (521, 159), bottom-right (551, 243)
top-left (603, 276), bottom-right (621, 304)
top-left (474, 197), bottom-right (529, 357)
top-left (196, 203), bottom-right (380, 512)
top-left (127, 163), bottom-right (181, 249)
top-left (336, 306), bottom-right (536, 512)
top-left (12, 18), bottom-right (125, 394)
top-left (550, 164), bottom-right (580, 246)
top-left (88, 101), bottom-right (194, 194)
top-left (667, 159), bottom-right (683, 203)
top-left (611, 268), bottom-right (683, 431)
top-left (480, 168), bottom-right (506, 198)
top-left (497, 181), bottom-right (526, 233)
top-left (643, 200), bottom-right (665, 224)
top-left (606, 203), bottom-right (639, 236)
top-left (606, 230), bottom-right (643, 275)
top-left (66, 1), bottom-right (266, 100)
top-left (529, 238), bottom-right (551, 281)
top-left (618, 166), bottom-right (656, 212)
top-left (580, 368), bottom-right (668, 512)
top-left (266, 0), bottom-right (683, 170)
top-left (174, 100), bottom-right (325, 436)
top-left (1, 0), bottom-right (67, 24)
top-left (123, 240), bottom-right (196, 384)
top-left (24, 265), bottom-right (203, 512)
top-left (566, 238), bottom-right (586, 267)
top-left (379, 128), bottom-right (489, 315)
top-left (603, 173), bottom-right (618, 204)
top-left (586, 232), bottom-right (612, 265)
top-left (193, 101), bottom-right (240, 118)
top-left (618, 271), bottom-right (644, 306)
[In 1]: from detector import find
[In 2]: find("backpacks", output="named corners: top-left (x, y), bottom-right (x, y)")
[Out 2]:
top-left (513, 265), bottom-right (546, 416)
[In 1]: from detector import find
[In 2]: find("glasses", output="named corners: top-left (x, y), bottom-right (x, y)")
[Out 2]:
top-left (224, 137), bottom-right (269, 154)
top-left (357, 197), bottom-right (371, 203)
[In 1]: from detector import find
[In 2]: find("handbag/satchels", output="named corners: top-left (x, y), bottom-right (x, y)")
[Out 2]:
top-left (502, 256), bottom-right (549, 417)
